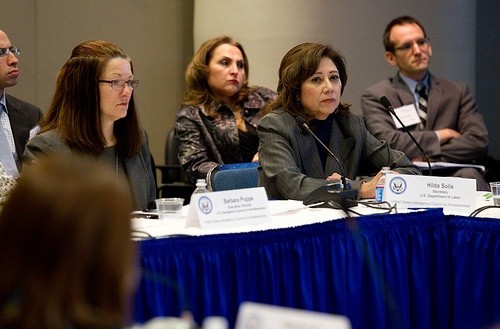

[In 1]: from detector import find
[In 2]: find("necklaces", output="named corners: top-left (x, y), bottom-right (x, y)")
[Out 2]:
top-left (237, 116), bottom-right (243, 128)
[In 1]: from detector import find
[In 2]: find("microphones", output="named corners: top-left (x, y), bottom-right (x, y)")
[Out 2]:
top-left (296, 115), bottom-right (359, 208)
top-left (380, 96), bottom-right (432, 176)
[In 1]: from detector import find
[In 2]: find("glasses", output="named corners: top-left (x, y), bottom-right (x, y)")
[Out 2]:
top-left (0, 47), bottom-right (21, 57)
top-left (99, 79), bottom-right (140, 90)
top-left (396, 40), bottom-right (426, 51)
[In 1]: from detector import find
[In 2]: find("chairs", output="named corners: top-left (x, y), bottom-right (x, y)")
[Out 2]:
top-left (149, 127), bottom-right (260, 205)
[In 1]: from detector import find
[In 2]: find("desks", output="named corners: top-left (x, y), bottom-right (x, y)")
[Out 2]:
top-left (130, 200), bottom-right (450, 329)
top-left (444, 191), bottom-right (500, 329)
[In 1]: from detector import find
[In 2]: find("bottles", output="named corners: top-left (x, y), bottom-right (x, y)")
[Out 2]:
top-left (190, 179), bottom-right (209, 205)
top-left (375, 167), bottom-right (390, 208)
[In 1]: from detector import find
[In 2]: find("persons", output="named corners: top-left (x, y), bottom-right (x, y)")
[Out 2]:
top-left (21, 40), bottom-right (158, 218)
top-left (255, 42), bottom-right (423, 202)
top-left (361, 14), bottom-right (492, 193)
top-left (173, 35), bottom-right (279, 183)
top-left (0, 153), bottom-right (137, 329)
top-left (0, 29), bottom-right (45, 207)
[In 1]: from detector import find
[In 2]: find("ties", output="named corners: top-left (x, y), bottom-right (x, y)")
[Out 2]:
top-left (0, 103), bottom-right (21, 173)
top-left (414, 84), bottom-right (429, 127)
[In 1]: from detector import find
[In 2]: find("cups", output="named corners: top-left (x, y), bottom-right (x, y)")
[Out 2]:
top-left (155, 197), bottom-right (185, 221)
top-left (490, 182), bottom-right (500, 206)
